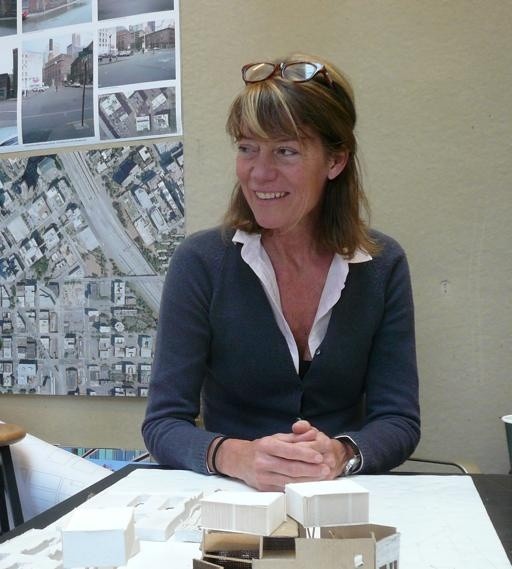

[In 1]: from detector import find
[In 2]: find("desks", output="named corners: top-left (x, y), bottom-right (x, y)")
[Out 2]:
top-left (2, 462), bottom-right (512, 569)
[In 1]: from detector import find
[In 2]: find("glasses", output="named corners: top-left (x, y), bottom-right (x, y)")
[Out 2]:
top-left (242, 61), bottom-right (339, 94)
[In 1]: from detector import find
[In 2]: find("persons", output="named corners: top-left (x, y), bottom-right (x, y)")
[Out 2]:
top-left (139, 53), bottom-right (422, 493)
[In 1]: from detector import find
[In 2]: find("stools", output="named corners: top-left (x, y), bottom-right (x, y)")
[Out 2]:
top-left (2, 422), bottom-right (28, 531)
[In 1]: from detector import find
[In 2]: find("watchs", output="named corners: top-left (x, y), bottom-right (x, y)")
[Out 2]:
top-left (342, 439), bottom-right (363, 477)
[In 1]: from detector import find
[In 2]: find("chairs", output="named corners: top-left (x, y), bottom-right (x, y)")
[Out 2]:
top-left (131, 451), bottom-right (464, 473)
